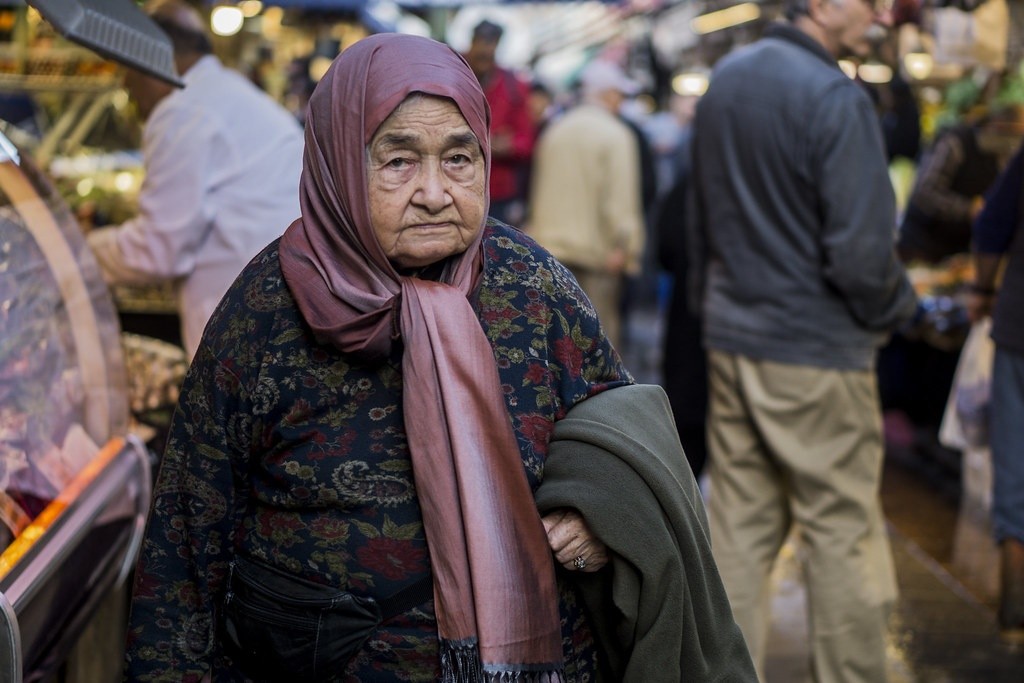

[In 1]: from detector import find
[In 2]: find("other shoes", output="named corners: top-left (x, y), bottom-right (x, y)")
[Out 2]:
top-left (998, 538), bottom-right (1024, 629)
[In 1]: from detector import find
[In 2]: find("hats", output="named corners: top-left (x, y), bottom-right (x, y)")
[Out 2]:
top-left (982, 101), bottom-right (1024, 134)
top-left (581, 59), bottom-right (641, 94)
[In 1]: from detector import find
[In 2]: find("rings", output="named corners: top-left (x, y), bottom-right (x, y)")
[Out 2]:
top-left (574, 556), bottom-right (586, 569)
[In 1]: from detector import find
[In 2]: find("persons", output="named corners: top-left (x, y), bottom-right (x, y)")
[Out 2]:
top-left (660, 0), bottom-right (924, 683)
top-left (528, 56), bottom-right (712, 384)
top-left (121, 32), bottom-right (764, 683)
top-left (898, 89), bottom-right (1023, 267)
top-left (464, 20), bottom-right (540, 222)
top-left (984, 138), bottom-right (1024, 651)
top-left (87, 3), bottom-right (309, 381)
